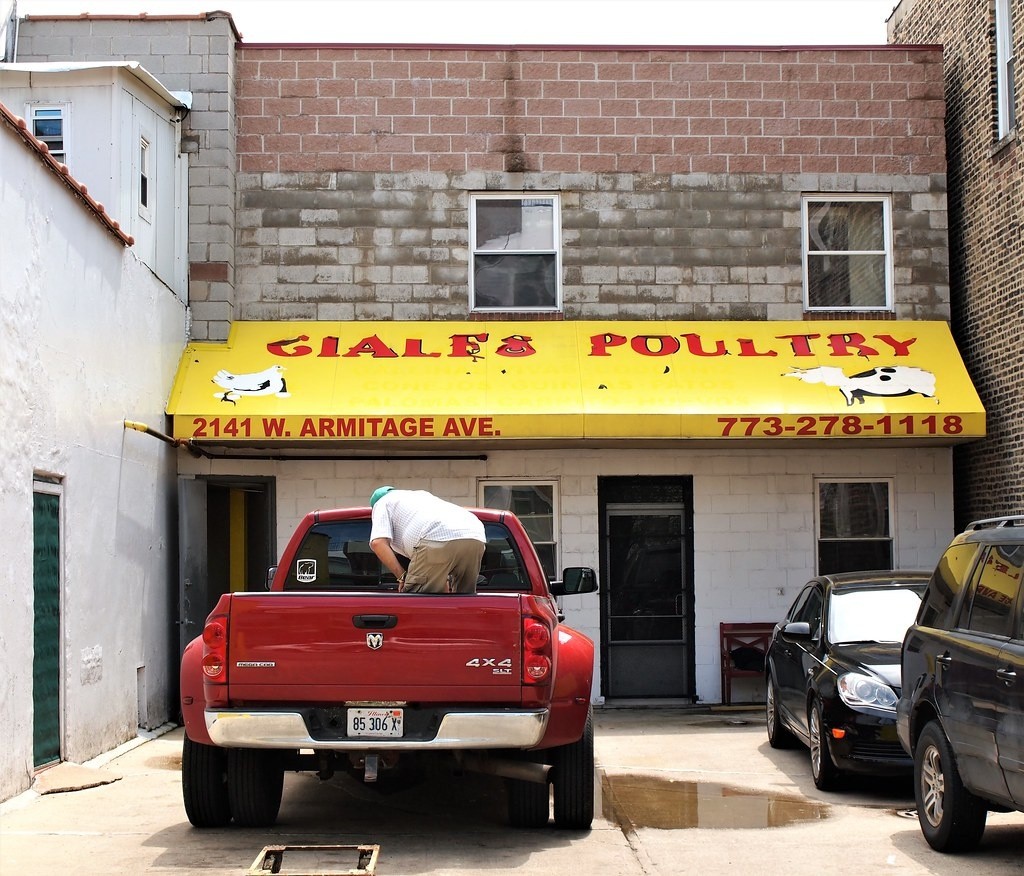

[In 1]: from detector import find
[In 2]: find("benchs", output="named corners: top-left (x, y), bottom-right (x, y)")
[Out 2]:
top-left (718, 620), bottom-right (779, 706)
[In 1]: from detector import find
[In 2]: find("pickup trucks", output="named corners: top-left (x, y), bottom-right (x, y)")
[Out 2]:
top-left (180, 506), bottom-right (598, 831)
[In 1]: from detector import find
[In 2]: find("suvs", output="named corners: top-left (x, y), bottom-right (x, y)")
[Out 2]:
top-left (896, 515), bottom-right (1024, 855)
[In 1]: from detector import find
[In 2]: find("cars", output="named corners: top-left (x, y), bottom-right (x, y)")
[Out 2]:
top-left (764, 570), bottom-right (935, 790)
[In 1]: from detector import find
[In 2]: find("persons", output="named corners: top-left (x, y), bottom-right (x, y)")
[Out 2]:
top-left (366, 484), bottom-right (487, 595)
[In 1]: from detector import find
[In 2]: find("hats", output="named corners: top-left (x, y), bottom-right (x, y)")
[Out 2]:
top-left (370, 486), bottom-right (395, 508)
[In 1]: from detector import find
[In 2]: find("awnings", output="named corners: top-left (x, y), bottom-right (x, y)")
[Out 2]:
top-left (169, 317), bottom-right (990, 449)
